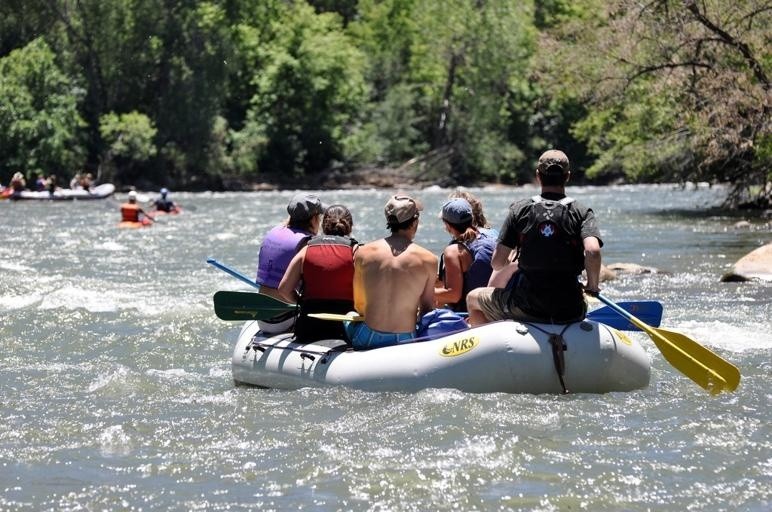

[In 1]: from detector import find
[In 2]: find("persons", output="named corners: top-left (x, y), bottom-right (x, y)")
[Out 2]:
top-left (432, 198), bottom-right (499, 316)
top-left (10, 167), bottom-right (93, 193)
top-left (149, 187), bottom-right (177, 212)
top-left (466, 149), bottom-right (605, 326)
top-left (343, 192), bottom-right (441, 353)
top-left (277, 205), bottom-right (364, 346)
top-left (432, 187), bottom-right (501, 290)
top-left (254, 191), bottom-right (329, 337)
top-left (119, 190), bottom-right (156, 223)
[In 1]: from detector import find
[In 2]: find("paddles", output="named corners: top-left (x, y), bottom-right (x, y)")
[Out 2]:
top-left (307, 313), bottom-right (364, 322)
top-left (213, 291), bottom-right (299, 321)
top-left (455, 301), bottom-right (663, 332)
top-left (582, 283), bottom-right (740, 396)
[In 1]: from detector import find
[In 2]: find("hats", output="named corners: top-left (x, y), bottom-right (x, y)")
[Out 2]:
top-left (287, 193), bottom-right (330, 218)
top-left (538, 150), bottom-right (570, 176)
top-left (438, 198), bottom-right (473, 224)
top-left (385, 194), bottom-right (423, 223)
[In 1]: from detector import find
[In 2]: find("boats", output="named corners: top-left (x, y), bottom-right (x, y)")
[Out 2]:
top-left (231, 288), bottom-right (650, 394)
top-left (13, 181), bottom-right (117, 200)
top-left (118, 202), bottom-right (183, 228)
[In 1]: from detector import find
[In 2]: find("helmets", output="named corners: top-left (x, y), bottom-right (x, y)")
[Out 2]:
top-left (161, 188), bottom-right (168, 194)
top-left (128, 191), bottom-right (137, 200)
